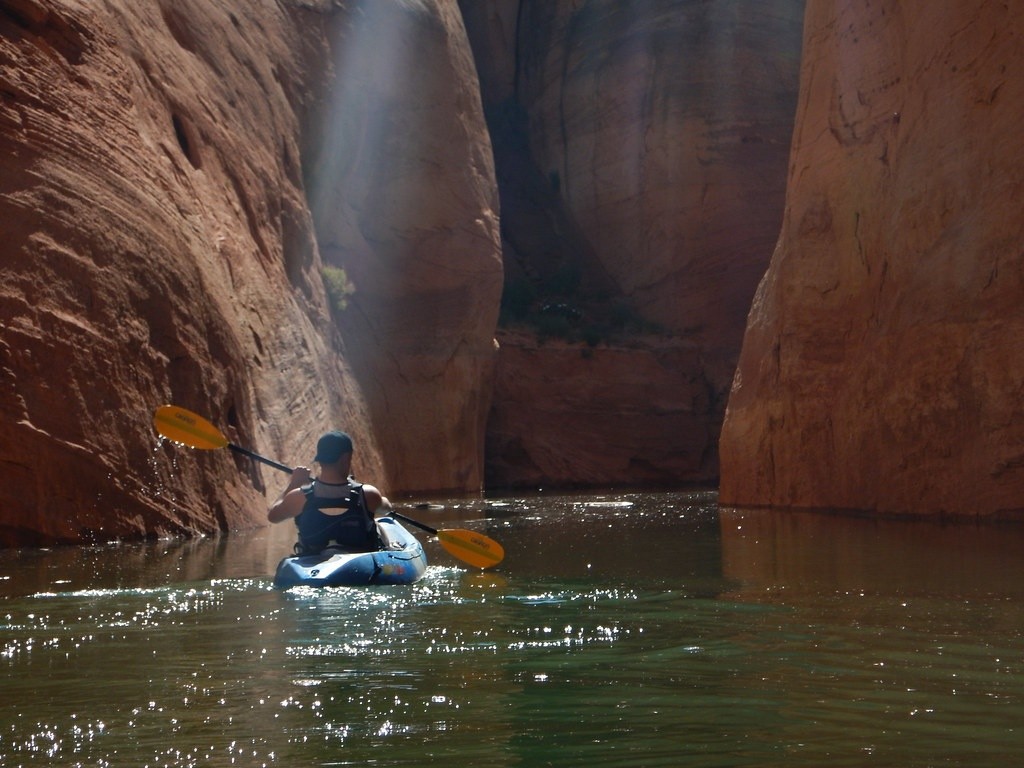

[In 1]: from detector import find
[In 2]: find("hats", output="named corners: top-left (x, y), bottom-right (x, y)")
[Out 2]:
top-left (314, 431), bottom-right (353, 463)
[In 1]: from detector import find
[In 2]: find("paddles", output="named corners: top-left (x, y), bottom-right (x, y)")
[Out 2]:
top-left (151, 405), bottom-right (506, 571)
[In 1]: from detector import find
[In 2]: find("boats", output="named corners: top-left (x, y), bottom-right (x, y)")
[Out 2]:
top-left (273, 516), bottom-right (431, 592)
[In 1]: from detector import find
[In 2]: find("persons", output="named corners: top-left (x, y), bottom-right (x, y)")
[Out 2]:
top-left (267, 431), bottom-right (393, 558)
